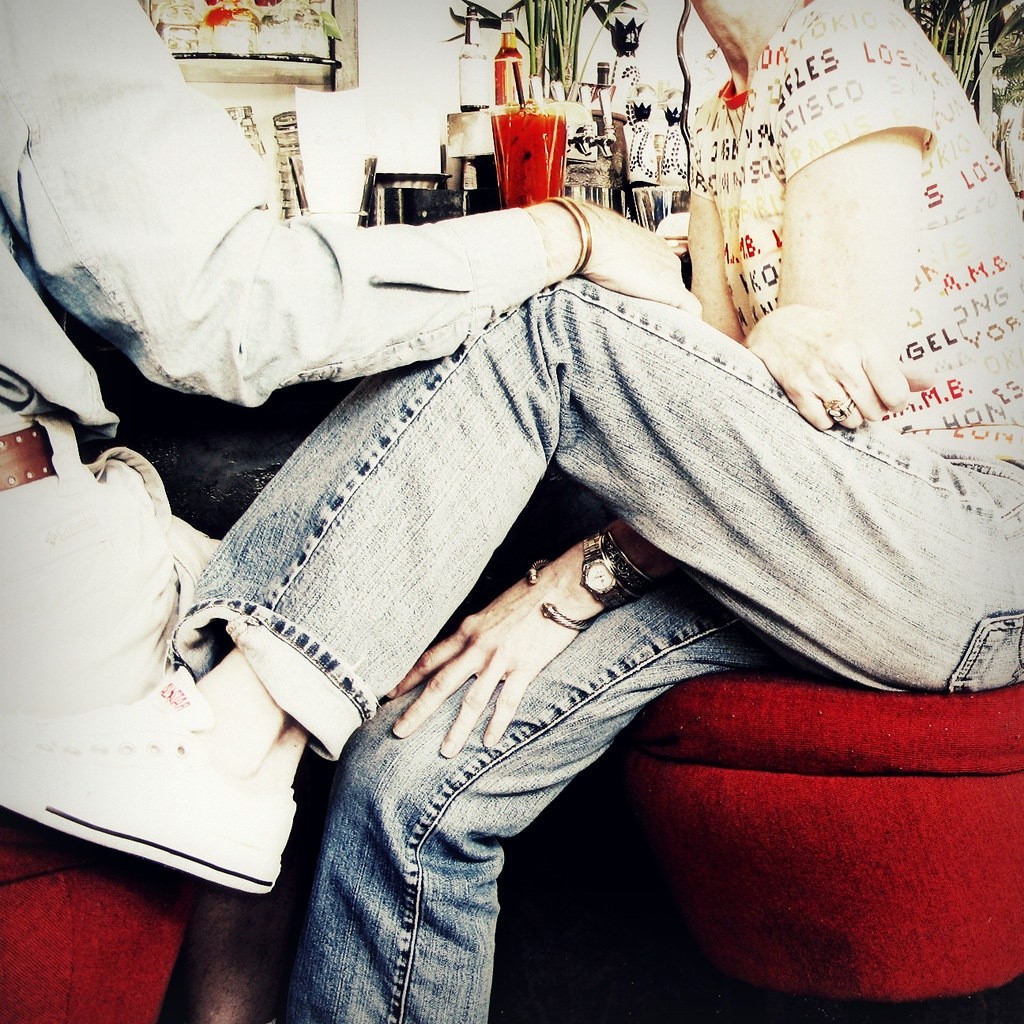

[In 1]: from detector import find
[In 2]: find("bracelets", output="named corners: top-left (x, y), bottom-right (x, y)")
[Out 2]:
top-left (525, 558), bottom-right (595, 631)
top-left (543, 196), bottom-right (593, 276)
top-left (600, 531), bottom-right (657, 598)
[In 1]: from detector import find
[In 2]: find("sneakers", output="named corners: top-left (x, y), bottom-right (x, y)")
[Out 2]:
top-left (0, 665), bottom-right (298, 894)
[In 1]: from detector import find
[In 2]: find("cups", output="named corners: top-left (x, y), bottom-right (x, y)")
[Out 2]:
top-left (491, 104), bottom-right (567, 208)
top-left (631, 186), bottom-right (690, 232)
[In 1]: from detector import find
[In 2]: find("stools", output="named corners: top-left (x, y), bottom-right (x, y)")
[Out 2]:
top-left (618, 671), bottom-right (1023, 1003)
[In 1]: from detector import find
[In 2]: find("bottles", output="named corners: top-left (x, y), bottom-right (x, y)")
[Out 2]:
top-left (591, 61), bottom-right (610, 103)
top-left (494, 13), bottom-right (524, 107)
top-left (459, 6), bottom-right (490, 112)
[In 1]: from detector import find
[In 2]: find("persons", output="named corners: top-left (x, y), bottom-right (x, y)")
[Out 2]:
top-left (0, 0), bottom-right (1024, 1024)
top-left (0, 0), bottom-right (703, 410)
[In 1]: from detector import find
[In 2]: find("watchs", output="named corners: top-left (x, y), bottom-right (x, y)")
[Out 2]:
top-left (579, 530), bottom-right (628, 610)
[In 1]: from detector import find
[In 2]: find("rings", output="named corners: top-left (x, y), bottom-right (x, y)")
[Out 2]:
top-left (822, 398), bottom-right (856, 423)
